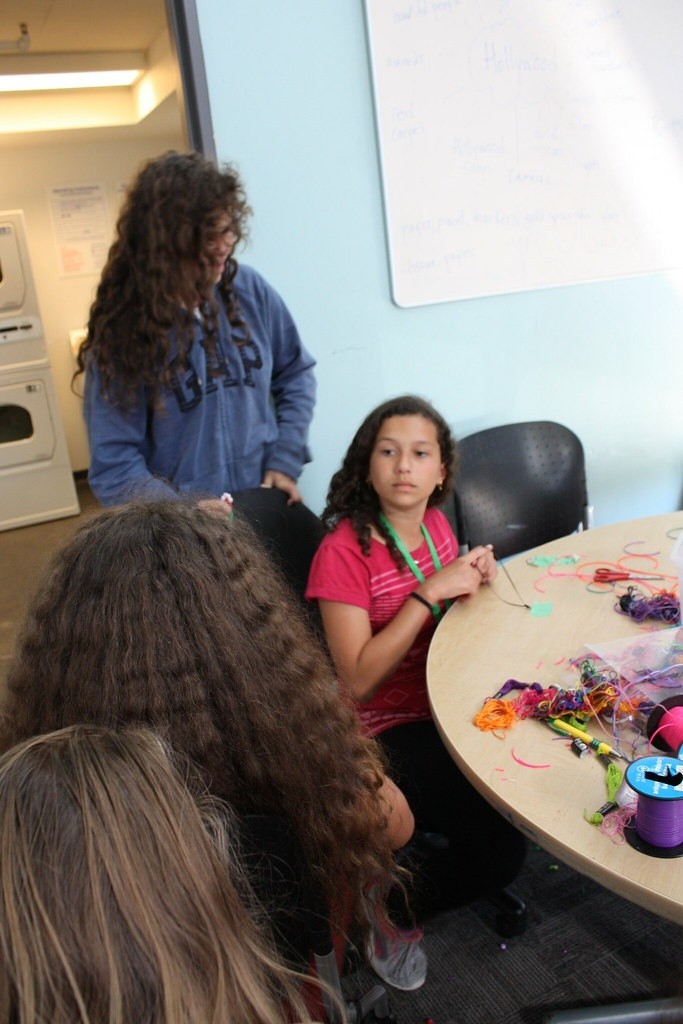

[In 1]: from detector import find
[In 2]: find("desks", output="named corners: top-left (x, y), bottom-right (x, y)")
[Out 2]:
top-left (425, 511), bottom-right (683, 1024)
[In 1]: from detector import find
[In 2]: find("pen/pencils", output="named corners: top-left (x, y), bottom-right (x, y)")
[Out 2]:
top-left (544, 716), bottom-right (623, 759)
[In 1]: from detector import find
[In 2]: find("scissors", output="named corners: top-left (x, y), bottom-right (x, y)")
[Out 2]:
top-left (594, 568), bottom-right (665, 583)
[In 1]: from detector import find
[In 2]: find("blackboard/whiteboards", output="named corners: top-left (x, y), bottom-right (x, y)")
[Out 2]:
top-left (364, 0), bottom-right (683, 310)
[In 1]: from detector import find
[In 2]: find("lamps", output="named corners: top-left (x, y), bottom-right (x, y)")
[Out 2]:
top-left (0, 48), bottom-right (148, 91)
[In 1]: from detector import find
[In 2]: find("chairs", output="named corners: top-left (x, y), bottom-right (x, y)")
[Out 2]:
top-left (225, 486), bottom-right (334, 683)
top-left (436, 421), bottom-right (593, 558)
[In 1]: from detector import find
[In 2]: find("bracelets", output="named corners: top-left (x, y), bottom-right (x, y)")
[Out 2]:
top-left (410, 592), bottom-right (440, 617)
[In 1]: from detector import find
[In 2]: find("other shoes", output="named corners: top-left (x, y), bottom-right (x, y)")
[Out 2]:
top-left (367, 932), bottom-right (428, 991)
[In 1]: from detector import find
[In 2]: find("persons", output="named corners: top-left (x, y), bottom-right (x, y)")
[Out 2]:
top-left (69, 150), bottom-right (316, 525)
top-left (1, 498), bottom-right (406, 1024)
top-left (304, 394), bottom-right (499, 992)
